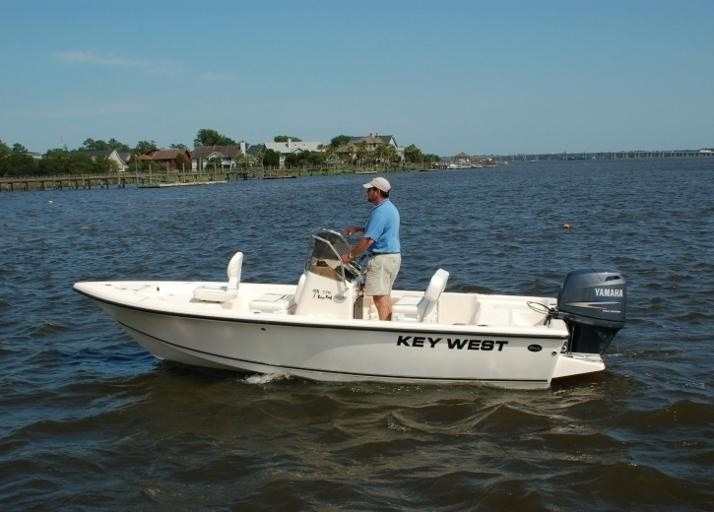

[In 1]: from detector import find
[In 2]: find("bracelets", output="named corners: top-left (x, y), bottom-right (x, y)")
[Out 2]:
top-left (348, 252), bottom-right (354, 260)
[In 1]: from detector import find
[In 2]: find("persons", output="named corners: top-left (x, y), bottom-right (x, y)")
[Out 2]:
top-left (340, 177), bottom-right (402, 321)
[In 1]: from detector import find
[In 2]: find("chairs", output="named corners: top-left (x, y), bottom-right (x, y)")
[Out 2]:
top-left (249, 273), bottom-right (306, 315)
top-left (392, 268), bottom-right (449, 324)
top-left (193, 251), bottom-right (243, 309)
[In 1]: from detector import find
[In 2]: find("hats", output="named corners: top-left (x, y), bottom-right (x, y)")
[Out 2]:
top-left (362, 178), bottom-right (391, 193)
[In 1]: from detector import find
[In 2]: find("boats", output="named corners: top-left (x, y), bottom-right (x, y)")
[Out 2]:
top-left (73, 229), bottom-right (626, 390)
